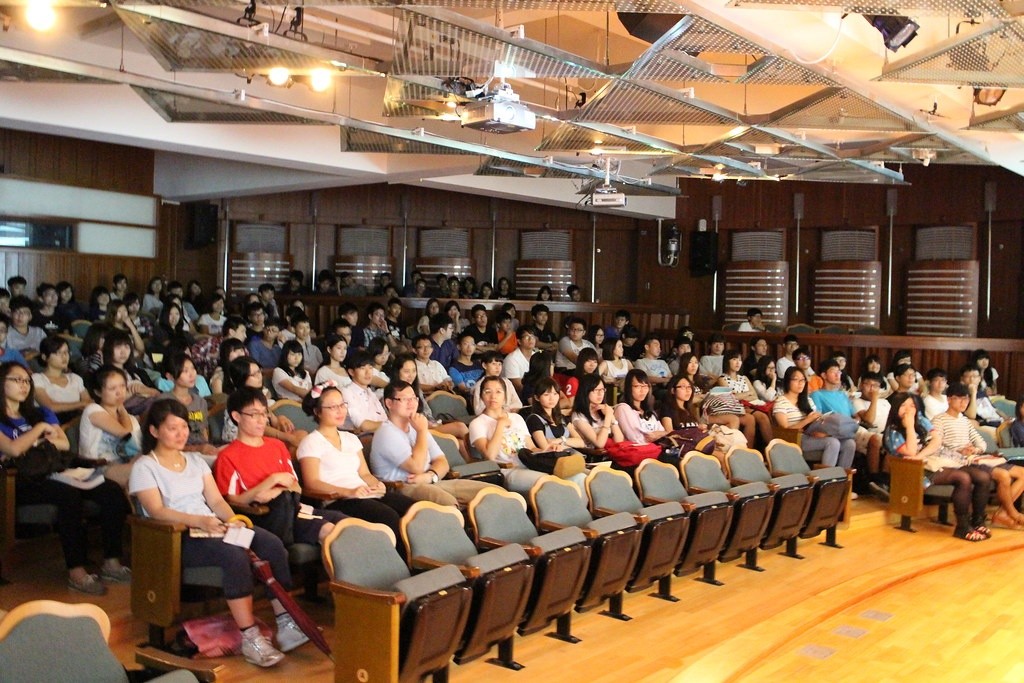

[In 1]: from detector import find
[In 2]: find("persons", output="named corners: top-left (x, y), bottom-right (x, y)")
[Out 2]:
top-left (0, 272), bottom-right (1024, 669)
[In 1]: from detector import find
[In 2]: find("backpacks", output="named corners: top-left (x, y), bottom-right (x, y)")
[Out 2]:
top-left (805, 409), bottom-right (859, 442)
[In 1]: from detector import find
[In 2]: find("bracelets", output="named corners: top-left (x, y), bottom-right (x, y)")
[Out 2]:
top-left (763, 326), bottom-right (768, 332)
top-left (560, 435), bottom-right (568, 442)
top-left (601, 425), bottom-right (611, 434)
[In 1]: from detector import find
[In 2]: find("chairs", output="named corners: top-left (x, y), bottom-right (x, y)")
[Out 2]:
top-left (0, 287), bottom-right (1024, 683)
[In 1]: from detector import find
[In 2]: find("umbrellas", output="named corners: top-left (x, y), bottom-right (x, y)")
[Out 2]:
top-left (227, 514), bottom-right (336, 663)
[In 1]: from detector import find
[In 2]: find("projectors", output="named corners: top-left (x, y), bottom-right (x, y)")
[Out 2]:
top-left (591, 193), bottom-right (627, 207)
top-left (461, 100), bottom-right (536, 134)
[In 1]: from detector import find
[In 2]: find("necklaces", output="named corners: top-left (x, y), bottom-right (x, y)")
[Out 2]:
top-left (158, 449), bottom-right (184, 469)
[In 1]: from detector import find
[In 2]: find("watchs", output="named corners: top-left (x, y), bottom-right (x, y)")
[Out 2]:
top-left (425, 469), bottom-right (439, 484)
top-left (611, 420), bottom-right (619, 426)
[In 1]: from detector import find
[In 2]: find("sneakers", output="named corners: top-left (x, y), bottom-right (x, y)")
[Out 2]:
top-left (238, 627), bottom-right (284, 668)
top-left (273, 612), bottom-right (326, 653)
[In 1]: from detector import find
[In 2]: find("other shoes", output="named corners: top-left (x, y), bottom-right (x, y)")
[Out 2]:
top-left (850, 491), bottom-right (859, 500)
top-left (869, 477), bottom-right (890, 503)
top-left (992, 513), bottom-right (1024, 529)
top-left (67, 574), bottom-right (107, 597)
top-left (953, 525), bottom-right (992, 541)
top-left (98, 566), bottom-right (134, 584)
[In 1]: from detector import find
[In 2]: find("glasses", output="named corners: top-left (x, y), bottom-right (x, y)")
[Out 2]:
top-left (6, 377), bottom-right (31, 385)
top-left (240, 312), bottom-right (695, 418)
top-left (795, 356), bottom-right (811, 360)
top-left (827, 369), bottom-right (843, 375)
top-left (791, 377), bottom-right (807, 383)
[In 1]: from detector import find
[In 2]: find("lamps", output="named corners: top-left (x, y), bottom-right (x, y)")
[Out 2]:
top-left (283, 0), bottom-right (334, 93)
top-left (850, 7), bottom-right (921, 52)
top-left (223, 0), bottom-right (294, 93)
top-left (946, 10), bottom-right (1009, 109)
top-left (661, 222), bottom-right (682, 267)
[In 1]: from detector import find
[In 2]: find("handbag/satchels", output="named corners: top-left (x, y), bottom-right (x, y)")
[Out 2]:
top-left (177, 609), bottom-right (274, 657)
top-left (518, 447), bottom-right (586, 479)
top-left (605, 436), bottom-right (660, 468)
top-left (741, 397), bottom-right (775, 412)
top-left (703, 392), bottom-right (746, 416)
top-left (656, 426), bottom-right (715, 459)
top-left (911, 442), bottom-right (967, 471)
top-left (707, 424), bottom-right (748, 452)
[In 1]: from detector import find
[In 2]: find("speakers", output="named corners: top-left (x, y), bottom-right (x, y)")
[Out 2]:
top-left (183, 202), bottom-right (217, 251)
top-left (689, 231), bottom-right (719, 278)
